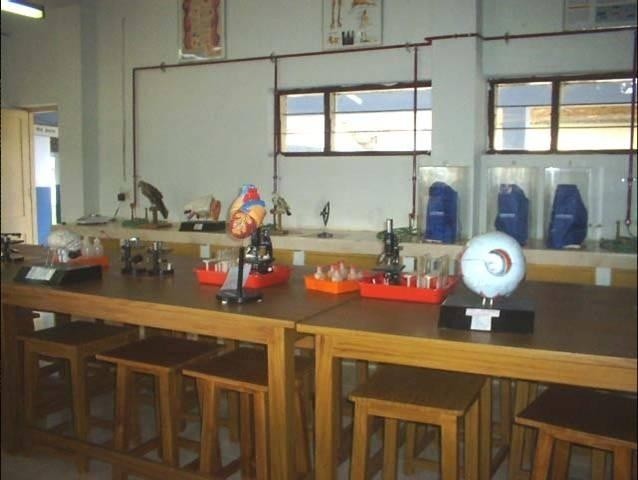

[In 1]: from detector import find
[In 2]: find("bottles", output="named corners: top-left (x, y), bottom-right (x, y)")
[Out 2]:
top-left (401, 254), bottom-right (449, 291)
top-left (81, 233), bottom-right (105, 258)
top-left (314, 258), bottom-right (370, 282)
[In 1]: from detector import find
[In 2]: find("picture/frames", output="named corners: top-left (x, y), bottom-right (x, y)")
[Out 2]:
top-left (321, 0), bottom-right (382, 50)
top-left (563, 0), bottom-right (638, 32)
top-left (176, 1), bottom-right (227, 63)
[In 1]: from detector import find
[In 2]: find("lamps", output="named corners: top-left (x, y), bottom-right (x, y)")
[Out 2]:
top-left (1, 0), bottom-right (46, 20)
